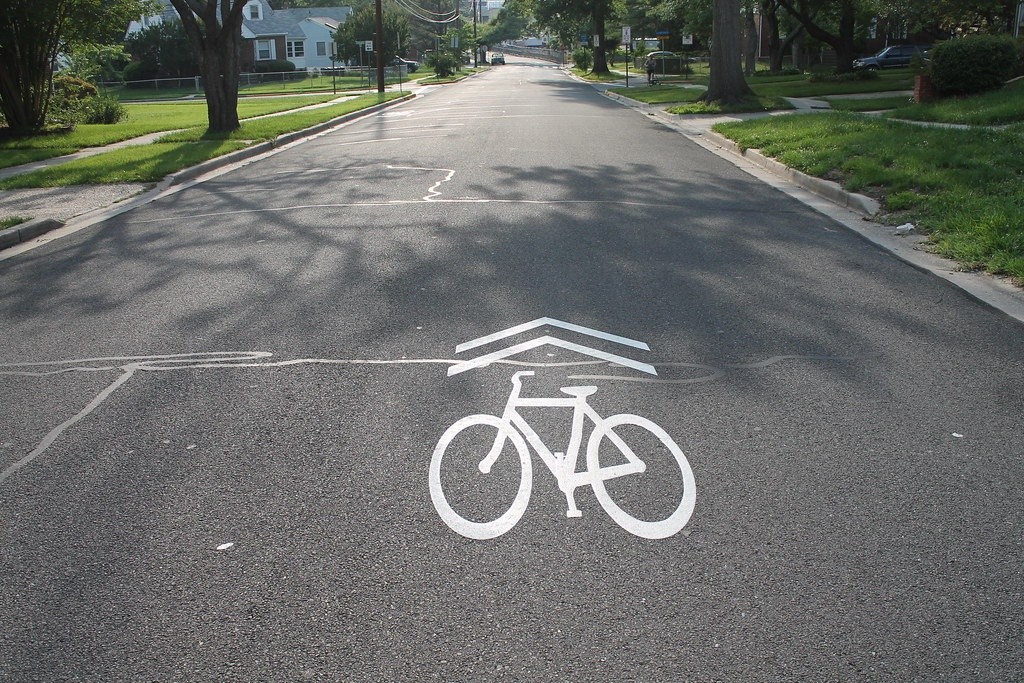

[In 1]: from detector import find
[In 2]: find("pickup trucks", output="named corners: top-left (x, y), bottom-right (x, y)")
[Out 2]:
top-left (491, 53), bottom-right (505, 65)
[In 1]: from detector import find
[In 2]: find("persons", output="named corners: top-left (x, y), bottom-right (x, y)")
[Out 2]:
top-left (644, 55), bottom-right (656, 76)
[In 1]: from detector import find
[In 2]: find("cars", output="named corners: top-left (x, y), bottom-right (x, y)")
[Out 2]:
top-left (644, 51), bottom-right (697, 63)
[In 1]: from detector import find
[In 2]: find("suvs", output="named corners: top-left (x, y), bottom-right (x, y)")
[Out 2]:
top-left (852, 44), bottom-right (925, 75)
top-left (391, 53), bottom-right (419, 73)
